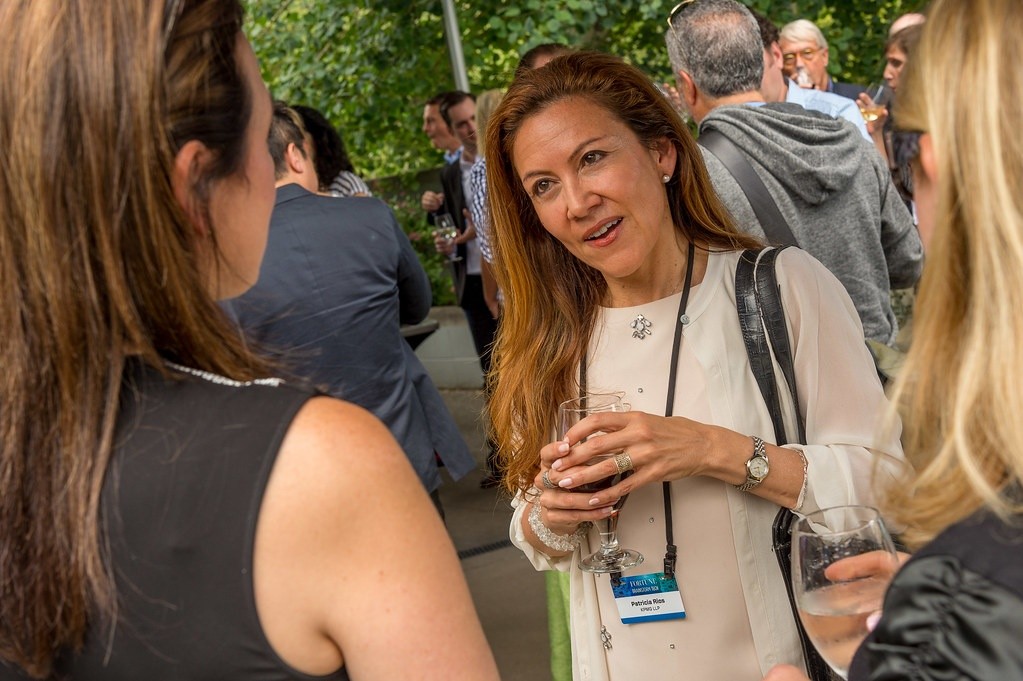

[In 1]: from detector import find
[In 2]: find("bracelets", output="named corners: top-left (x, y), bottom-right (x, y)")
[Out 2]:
top-left (527, 493), bottom-right (589, 552)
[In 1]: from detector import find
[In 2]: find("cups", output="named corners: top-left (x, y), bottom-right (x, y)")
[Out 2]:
top-left (652, 82), bottom-right (690, 122)
top-left (859, 82), bottom-right (892, 122)
top-left (792, 504), bottom-right (901, 679)
top-left (794, 65), bottom-right (816, 88)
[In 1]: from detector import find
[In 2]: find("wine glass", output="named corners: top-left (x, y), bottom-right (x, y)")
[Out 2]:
top-left (435, 214), bottom-right (462, 264)
top-left (558, 396), bottom-right (646, 572)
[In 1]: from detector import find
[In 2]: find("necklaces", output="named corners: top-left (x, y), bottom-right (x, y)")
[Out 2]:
top-left (609, 271), bottom-right (685, 307)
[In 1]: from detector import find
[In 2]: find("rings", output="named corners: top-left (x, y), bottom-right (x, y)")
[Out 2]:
top-left (614, 452), bottom-right (634, 475)
top-left (543, 469), bottom-right (558, 489)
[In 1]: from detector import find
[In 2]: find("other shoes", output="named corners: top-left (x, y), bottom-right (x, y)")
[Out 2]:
top-left (481, 474), bottom-right (503, 488)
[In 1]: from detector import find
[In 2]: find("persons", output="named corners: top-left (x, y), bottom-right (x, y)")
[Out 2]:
top-left (420, 44), bottom-right (570, 486)
top-left (485, 54), bottom-right (916, 681)
top-left (0, 0), bottom-right (499, 681)
top-left (214, 102), bottom-right (477, 525)
top-left (765, 0), bottom-right (1023, 681)
top-left (663, 0), bottom-right (928, 385)
top-left (293, 102), bottom-right (372, 197)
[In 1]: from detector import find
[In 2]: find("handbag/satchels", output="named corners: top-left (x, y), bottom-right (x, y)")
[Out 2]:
top-left (769, 503), bottom-right (907, 681)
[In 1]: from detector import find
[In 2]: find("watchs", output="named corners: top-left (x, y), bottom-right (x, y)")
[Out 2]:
top-left (735, 434), bottom-right (770, 492)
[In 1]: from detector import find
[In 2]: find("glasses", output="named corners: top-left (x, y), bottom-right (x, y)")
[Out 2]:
top-left (782, 45), bottom-right (825, 66)
top-left (878, 111), bottom-right (926, 200)
top-left (668, 0), bottom-right (694, 69)
